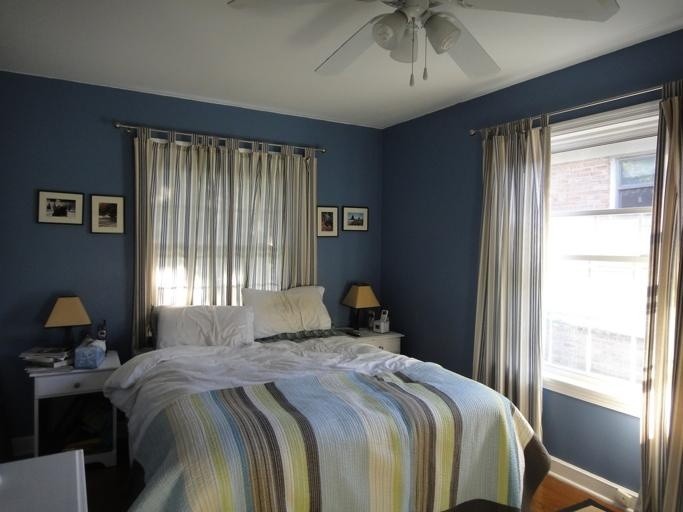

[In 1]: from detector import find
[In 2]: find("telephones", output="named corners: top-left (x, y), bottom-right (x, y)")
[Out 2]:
top-left (98, 320), bottom-right (108, 354)
top-left (372, 310), bottom-right (389, 333)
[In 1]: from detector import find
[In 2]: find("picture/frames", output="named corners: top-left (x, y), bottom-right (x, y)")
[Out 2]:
top-left (342, 206), bottom-right (368, 231)
top-left (318, 206), bottom-right (339, 237)
top-left (38, 190), bottom-right (85, 225)
top-left (91, 194), bottom-right (125, 234)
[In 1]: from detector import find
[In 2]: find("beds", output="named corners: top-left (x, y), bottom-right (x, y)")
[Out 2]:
top-left (103, 335), bottom-right (551, 511)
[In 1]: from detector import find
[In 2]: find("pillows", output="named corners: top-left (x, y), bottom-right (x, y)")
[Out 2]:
top-left (241, 286), bottom-right (332, 341)
top-left (157, 304), bottom-right (254, 350)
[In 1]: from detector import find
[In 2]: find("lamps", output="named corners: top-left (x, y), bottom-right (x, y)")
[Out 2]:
top-left (341, 284), bottom-right (381, 327)
top-left (43, 296), bottom-right (92, 350)
top-left (373, 1), bottom-right (461, 87)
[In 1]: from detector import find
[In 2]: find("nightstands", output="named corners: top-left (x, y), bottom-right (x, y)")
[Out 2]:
top-left (334, 327), bottom-right (405, 355)
top-left (28, 350), bottom-right (122, 468)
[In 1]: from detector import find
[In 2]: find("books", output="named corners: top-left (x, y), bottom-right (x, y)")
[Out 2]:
top-left (18, 347), bottom-right (73, 373)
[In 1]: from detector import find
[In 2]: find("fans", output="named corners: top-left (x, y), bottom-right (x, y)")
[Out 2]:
top-left (227, 0), bottom-right (620, 80)
